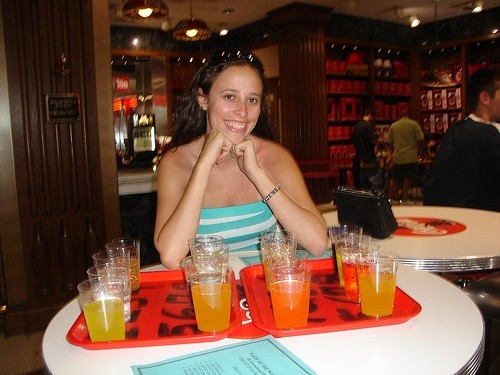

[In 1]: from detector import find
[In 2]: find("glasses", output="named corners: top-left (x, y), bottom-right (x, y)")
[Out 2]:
top-left (208, 49), bottom-right (264, 75)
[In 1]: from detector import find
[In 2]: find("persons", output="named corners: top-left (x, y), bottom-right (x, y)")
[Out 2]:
top-left (153, 48), bottom-right (329, 269)
top-left (388, 112), bottom-right (424, 200)
top-left (352, 110), bottom-right (382, 193)
top-left (423, 66), bottom-right (500, 214)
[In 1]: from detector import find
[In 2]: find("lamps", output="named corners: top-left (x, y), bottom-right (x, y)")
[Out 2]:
top-left (472, 0), bottom-right (484, 13)
top-left (122, 0), bottom-right (168, 21)
top-left (172, 0), bottom-right (211, 41)
top-left (409, 15), bottom-right (420, 28)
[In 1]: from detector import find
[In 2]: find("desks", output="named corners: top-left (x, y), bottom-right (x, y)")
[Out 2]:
top-left (41, 250), bottom-right (486, 374)
top-left (321, 205), bottom-right (498, 272)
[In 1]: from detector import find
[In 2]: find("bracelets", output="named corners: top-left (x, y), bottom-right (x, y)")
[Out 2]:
top-left (262, 185), bottom-right (281, 203)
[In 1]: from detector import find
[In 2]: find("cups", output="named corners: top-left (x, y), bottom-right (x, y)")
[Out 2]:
top-left (76, 238), bottom-right (141, 342)
top-left (179, 236), bottom-right (234, 332)
top-left (328, 224), bottom-right (400, 317)
top-left (259, 230), bottom-right (313, 327)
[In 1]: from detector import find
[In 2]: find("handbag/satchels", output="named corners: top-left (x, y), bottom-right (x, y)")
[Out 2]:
top-left (333, 188), bottom-right (398, 239)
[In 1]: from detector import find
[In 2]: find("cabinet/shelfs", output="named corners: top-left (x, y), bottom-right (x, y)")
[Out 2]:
top-left (325, 73), bottom-right (462, 144)
